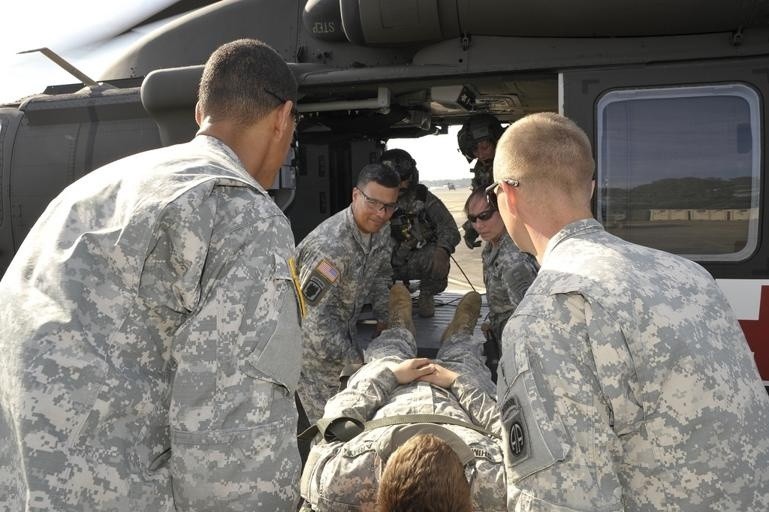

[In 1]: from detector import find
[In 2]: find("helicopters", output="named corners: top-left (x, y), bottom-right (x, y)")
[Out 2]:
top-left (0, 0), bottom-right (769, 396)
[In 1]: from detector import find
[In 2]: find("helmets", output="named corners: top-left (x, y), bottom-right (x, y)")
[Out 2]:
top-left (462, 114), bottom-right (506, 152)
top-left (378, 149), bottom-right (417, 183)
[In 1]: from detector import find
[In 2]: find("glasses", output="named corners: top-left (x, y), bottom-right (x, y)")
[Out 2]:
top-left (466, 207), bottom-right (499, 223)
top-left (265, 89), bottom-right (302, 126)
top-left (485, 176), bottom-right (518, 204)
top-left (356, 185), bottom-right (400, 213)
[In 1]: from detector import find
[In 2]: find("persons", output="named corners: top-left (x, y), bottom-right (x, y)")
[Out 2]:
top-left (376, 149), bottom-right (462, 320)
top-left (456, 115), bottom-right (510, 254)
top-left (296, 282), bottom-right (507, 511)
top-left (490, 109), bottom-right (769, 512)
top-left (293, 162), bottom-right (403, 474)
top-left (0, 36), bottom-right (307, 512)
top-left (465, 186), bottom-right (541, 387)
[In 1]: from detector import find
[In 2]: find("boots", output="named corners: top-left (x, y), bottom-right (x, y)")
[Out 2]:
top-left (387, 283), bottom-right (416, 340)
top-left (417, 293), bottom-right (436, 318)
top-left (440, 291), bottom-right (482, 347)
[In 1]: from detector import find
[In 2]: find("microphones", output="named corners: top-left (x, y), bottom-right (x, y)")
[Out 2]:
top-left (483, 158), bottom-right (495, 165)
top-left (398, 186), bottom-right (415, 193)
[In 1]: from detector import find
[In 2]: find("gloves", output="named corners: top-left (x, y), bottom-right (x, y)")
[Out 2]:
top-left (465, 225), bottom-right (482, 249)
top-left (424, 246), bottom-right (451, 281)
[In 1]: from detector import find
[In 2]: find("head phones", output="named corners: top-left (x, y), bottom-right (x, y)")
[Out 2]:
top-left (410, 159), bottom-right (420, 184)
